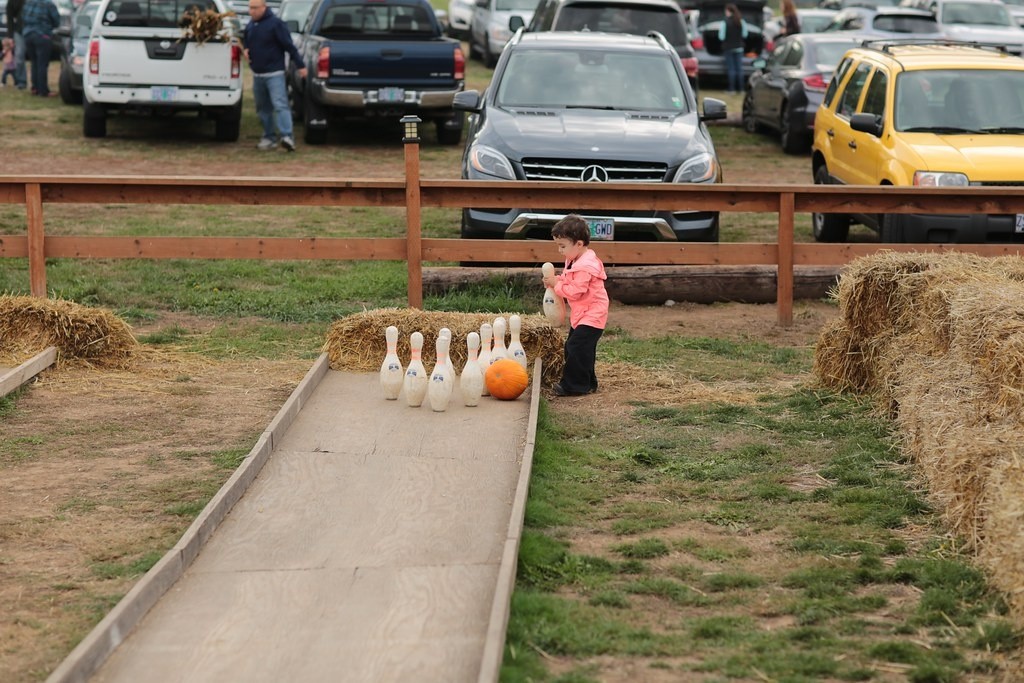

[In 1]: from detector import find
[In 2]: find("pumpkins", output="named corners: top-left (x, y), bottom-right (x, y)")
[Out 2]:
top-left (485, 359), bottom-right (528, 400)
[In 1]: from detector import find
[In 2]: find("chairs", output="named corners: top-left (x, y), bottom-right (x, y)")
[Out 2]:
top-left (182, 3), bottom-right (205, 27)
top-left (392, 11), bottom-right (413, 30)
top-left (118, 1), bottom-right (141, 16)
top-left (331, 12), bottom-right (352, 27)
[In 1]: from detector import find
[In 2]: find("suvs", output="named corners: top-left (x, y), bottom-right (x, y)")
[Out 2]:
top-left (453, 0), bottom-right (729, 267)
top-left (809, 39), bottom-right (1024, 244)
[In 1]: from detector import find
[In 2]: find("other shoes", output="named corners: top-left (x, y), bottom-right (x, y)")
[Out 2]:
top-left (256, 138), bottom-right (278, 149)
top-left (48, 91), bottom-right (59, 97)
top-left (31, 88), bottom-right (39, 94)
top-left (280, 136), bottom-right (296, 152)
top-left (553, 383), bottom-right (597, 396)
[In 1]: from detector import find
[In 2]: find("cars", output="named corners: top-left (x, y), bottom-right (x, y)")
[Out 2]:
top-left (681, 0), bottom-right (1023, 154)
top-left (446, 0), bottom-right (541, 69)
top-left (0, 0), bottom-right (311, 105)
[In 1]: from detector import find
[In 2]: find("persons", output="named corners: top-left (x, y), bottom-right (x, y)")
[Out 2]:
top-left (238, 0), bottom-right (308, 153)
top-left (541, 214), bottom-right (611, 396)
top-left (1, 0), bottom-right (60, 97)
top-left (698, 5), bottom-right (762, 96)
top-left (771, 0), bottom-right (801, 43)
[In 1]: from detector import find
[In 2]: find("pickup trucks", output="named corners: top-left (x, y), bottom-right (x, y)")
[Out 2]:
top-left (83, 0), bottom-right (248, 143)
top-left (286, 0), bottom-right (470, 143)
